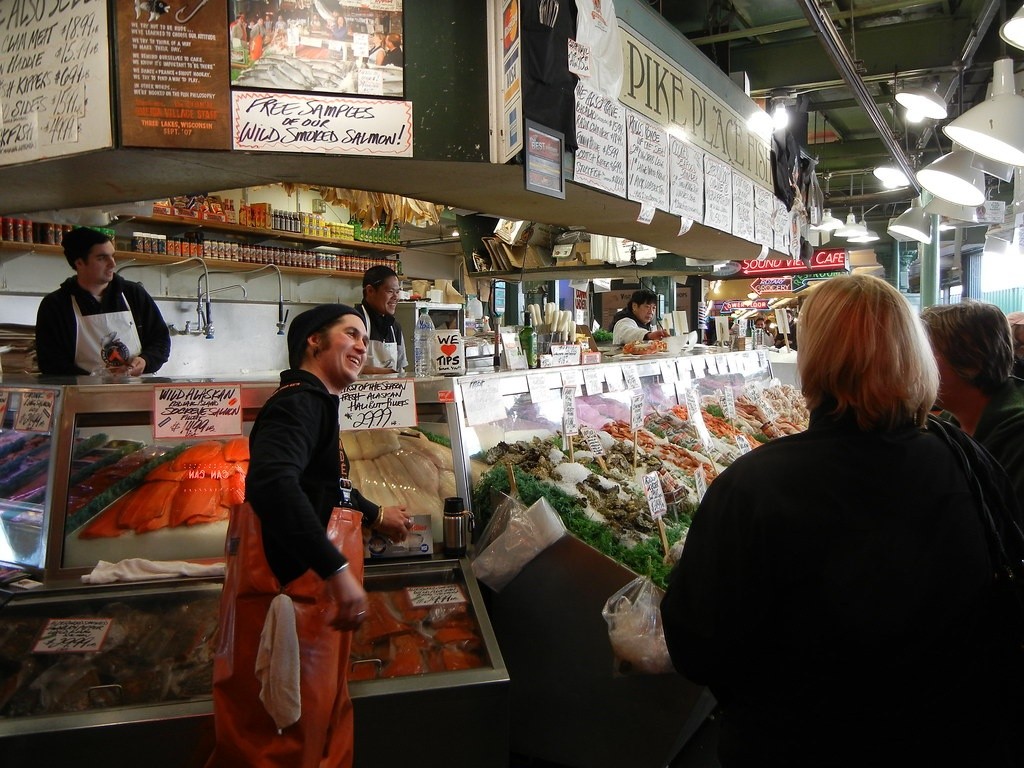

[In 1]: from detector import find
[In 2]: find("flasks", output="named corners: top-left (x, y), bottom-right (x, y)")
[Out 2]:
top-left (442, 497), bottom-right (475, 558)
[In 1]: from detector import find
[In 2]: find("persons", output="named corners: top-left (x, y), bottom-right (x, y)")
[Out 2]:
top-left (213, 301), bottom-right (413, 768)
top-left (352, 265), bottom-right (411, 377)
top-left (659, 276), bottom-right (1024, 768)
top-left (764, 319), bottom-right (776, 336)
top-left (33, 228), bottom-right (172, 379)
top-left (753, 316), bottom-right (773, 346)
top-left (775, 310), bottom-right (798, 350)
top-left (1004, 311), bottom-right (1024, 380)
top-left (914, 299), bottom-right (1024, 477)
top-left (608, 290), bottom-right (671, 347)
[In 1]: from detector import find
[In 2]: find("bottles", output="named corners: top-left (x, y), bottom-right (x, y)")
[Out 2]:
top-left (468, 296), bottom-right (484, 336)
top-left (1, 193), bottom-right (404, 278)
top-left (519, 311), bottom-right (538, 369)
top-left (414, 306), bottom-right (438, 378)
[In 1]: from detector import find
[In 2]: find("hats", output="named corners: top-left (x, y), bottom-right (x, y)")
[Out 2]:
top-left (288, 301), bottom-right (368, 370)
top-left (61, 226), bottom-right (109, 270)
top-left (363, 265), bottom-right (398, 288)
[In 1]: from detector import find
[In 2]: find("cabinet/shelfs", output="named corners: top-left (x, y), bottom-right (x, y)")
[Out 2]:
top-left (44, 382), bottom-right (475, 590)
top-left (0, 562), bottom-right (513, 739)
top-left (1, 382), bottom-right (50, 566)
top-left (0, 212), bottom-right (409, 281)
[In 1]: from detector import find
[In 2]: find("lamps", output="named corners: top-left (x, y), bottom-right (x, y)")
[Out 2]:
top-left (809, 178), bottom-right (844, 231)
top-left (894, 78), bottom-right (949, 123)
top-left (941, 2), bottom-right (1024, 169)
top-left (833, 174), bottom-right (869, 237)
top-left (914, 71), bottom-right (986, 206)
top-left (773, 102), bottom-right (788, 128)
top-left (846, 175), bottom-right (881, 243)
top-left (873, 163), bottom-right (910, 189)
top-left (887, 193), bottom-right (933, 245)
top-left (920, 197), bottom-right (983, 225)
top-left (998, 1), bottom-right (1024, 51)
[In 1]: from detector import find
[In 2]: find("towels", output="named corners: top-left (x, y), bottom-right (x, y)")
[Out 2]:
top-left (254, 594), bottom-right (301, 735)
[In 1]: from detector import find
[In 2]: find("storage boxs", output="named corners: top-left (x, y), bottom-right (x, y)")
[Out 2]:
top-left (454, 211), bottom-right (717, 275)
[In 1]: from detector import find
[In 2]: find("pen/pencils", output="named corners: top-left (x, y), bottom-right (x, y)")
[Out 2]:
top-left (656, 318), bottom-right (664, 331)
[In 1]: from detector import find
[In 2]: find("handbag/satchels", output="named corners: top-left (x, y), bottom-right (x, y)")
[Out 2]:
top-left (601, 569), bottom-right (677, 674)
